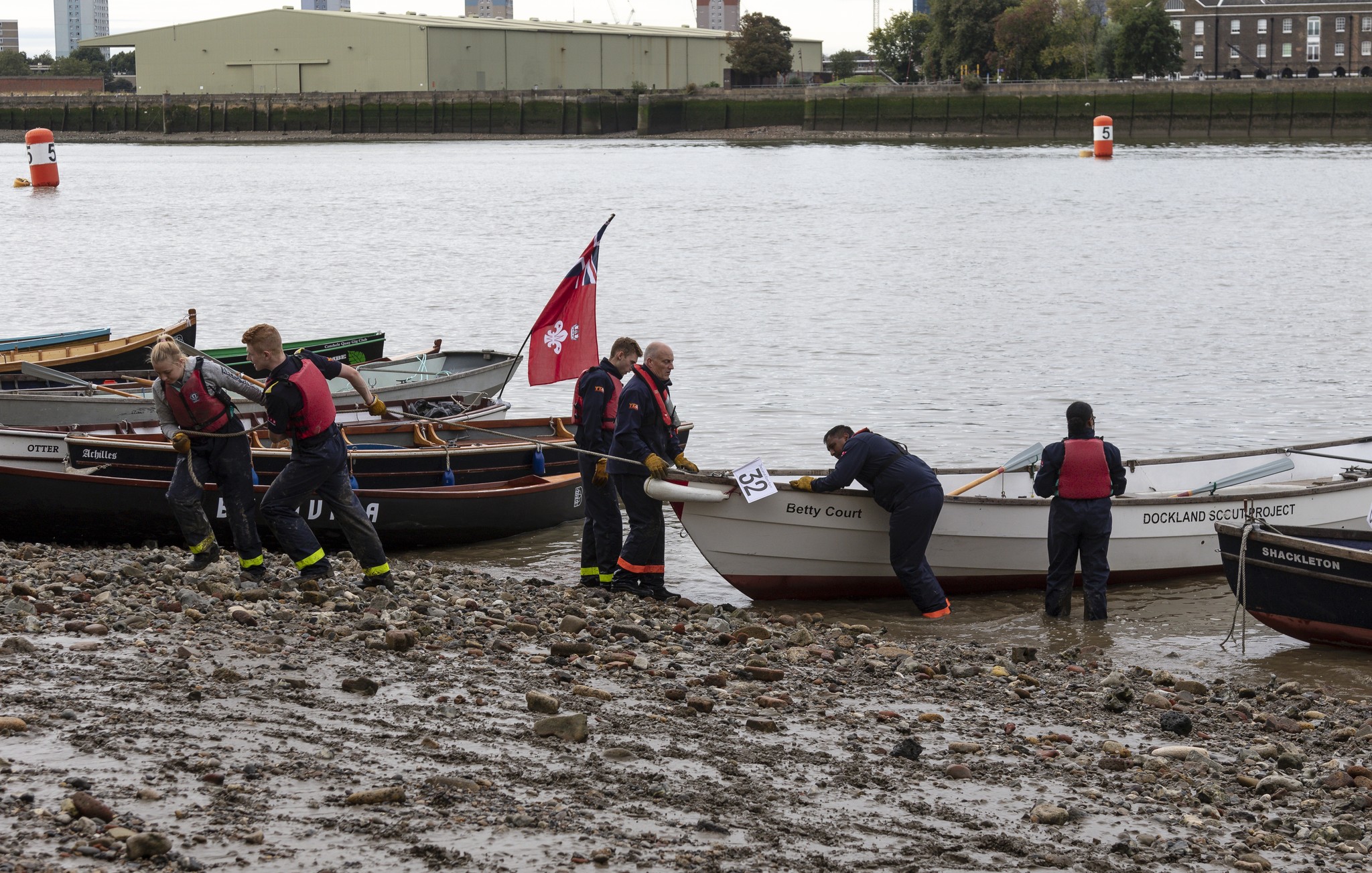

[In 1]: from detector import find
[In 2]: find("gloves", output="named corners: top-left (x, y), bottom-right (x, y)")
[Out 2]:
top-left (673, 454), bottom-right (700, 475)
top-left (172, 433), bottom-right (191, 453)
top-left (644, 453), bottom-right (670, 480)
top-left (363, 394), bottom-right (386, 415)
top-left (591, 458), bottom-right (609, 485)
top-left (789, 476), bottom-right (816, 493)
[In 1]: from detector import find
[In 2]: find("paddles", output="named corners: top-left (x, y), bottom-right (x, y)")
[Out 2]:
top-left (174, 339), bottom-right (266, 388)
top-left (1166, 457), bottom-right (1295, 498)
top-left (946, 442), bottom-right (1045, 495)
top-left (21, 362), bottom-right (146, 398)
top-left (121, 375), bottom-right (155, 385)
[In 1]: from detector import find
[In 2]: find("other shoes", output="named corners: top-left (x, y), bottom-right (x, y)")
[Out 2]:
top-left (183, 551), bottom-right (220, 571)
top-left (240, 571), bottom-right (263, 581)
top-left (287, 568), bottom-right (335, 583)
top-left (349, 580), bottom-right (396, 593)
top-left (640, 581), bottom-right (681, 601)
top-left (611, 582), bottom-right (655, 599)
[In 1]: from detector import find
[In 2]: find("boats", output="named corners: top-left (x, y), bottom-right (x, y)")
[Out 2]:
top-left (635, 436), bottom-right (1372, 605)
top-left (0, 309), bottom-right (591, 546)
top-left (1215, 499), bottom-right (1372, 652)
top-left (1, 327), bottom-right (112, 350)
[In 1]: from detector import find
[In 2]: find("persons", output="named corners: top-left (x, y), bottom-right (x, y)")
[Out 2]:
top-left (242, 325), bottom-right (393, 588)
top-left (570, 336), bottom-right (644, 594)
top-left (1034, 404), bottom-right (1127, 629)
top-left (151, 331), bottom-right (270, 579)
top-left (609, 343), bottom-right (703, 598)
top-left (789, 426), bottom-right (959, 622)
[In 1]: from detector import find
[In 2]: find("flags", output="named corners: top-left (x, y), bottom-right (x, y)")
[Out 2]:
top-left (528, 220), bottom-right (611, 386)
